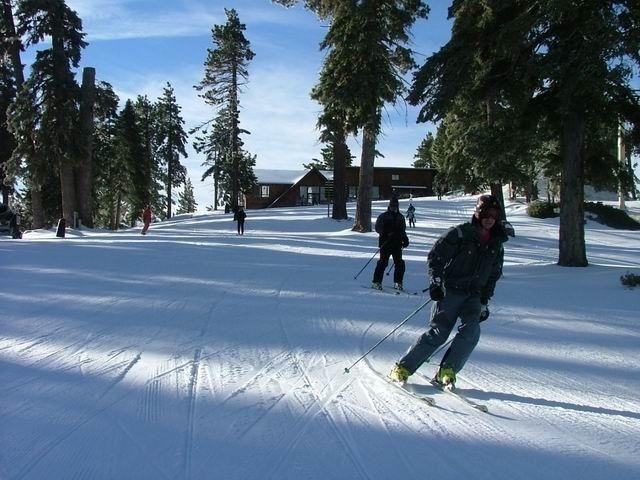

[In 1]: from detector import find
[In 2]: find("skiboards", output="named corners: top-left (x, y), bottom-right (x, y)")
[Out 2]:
top-left (369, 363), bottom-right (487, 413)
top-left (360, 285), bottom-right (417, 295)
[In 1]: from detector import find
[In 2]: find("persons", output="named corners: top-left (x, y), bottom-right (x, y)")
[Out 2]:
top-left (405, 202), bottom-right (416, 227)
top-left (140, 205), bottom-right (152, 235)
top-left (233, 206), bottom-right (247, 235)
top-left (391, 195), bottom-right (504, 392)
top-left (371, 197), bottom-right (410, 289)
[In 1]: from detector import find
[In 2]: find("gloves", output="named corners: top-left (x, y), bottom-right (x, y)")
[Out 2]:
top-left (428, 283), bottom-right (444, 301)
top-left (401, 236), bottom-right (409, 248)
top-left (479, 308), bottom-right (489, 321)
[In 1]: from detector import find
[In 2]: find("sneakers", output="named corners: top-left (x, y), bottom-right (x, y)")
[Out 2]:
top-left (372, 282), bottom-right (382, 290)
top-left (389, 363), bottom-right (412, 388)
top-left (394, 283), bottom-right (403, 290)
top-left (434, 368), bottom-right (455, 391)
